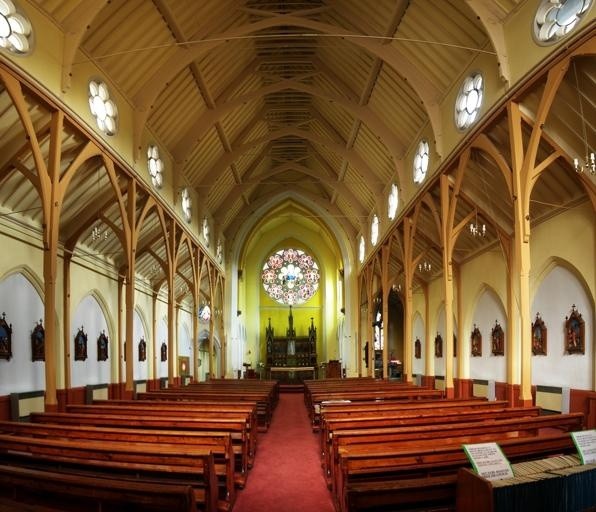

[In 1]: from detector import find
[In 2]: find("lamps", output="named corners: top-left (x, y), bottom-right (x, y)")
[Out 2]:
top-left (570, 55), bottom-right (596, 175)
top-left (469, 150), bottom-right (488, 240)
top-left (370, 202), bottom-right (433, 305)
top-left (91, 157), bottom-right (223, 317)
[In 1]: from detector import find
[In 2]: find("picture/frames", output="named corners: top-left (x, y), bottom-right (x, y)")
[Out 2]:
top-left (0, 313), bottom-right (167, 362)
top-left (415, 305), bottom-right (586, 359)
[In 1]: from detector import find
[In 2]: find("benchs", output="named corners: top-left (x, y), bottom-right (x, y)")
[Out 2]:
top-left (0, 378), bottom-right (280, 512)
top-left (303, 376), bottom-right (586, 512)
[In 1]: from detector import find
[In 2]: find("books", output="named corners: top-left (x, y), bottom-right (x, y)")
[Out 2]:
top-left (469, 452), bottom-right (596, 511)
